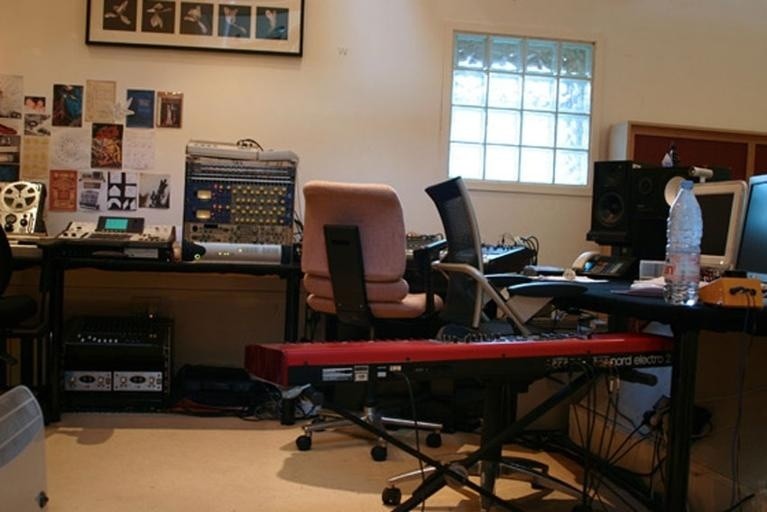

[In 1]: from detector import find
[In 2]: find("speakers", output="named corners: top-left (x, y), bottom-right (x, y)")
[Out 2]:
top-left (632, 166), bottom-right (680, 221)
top-left (591, 160), bottom-right (633, 232)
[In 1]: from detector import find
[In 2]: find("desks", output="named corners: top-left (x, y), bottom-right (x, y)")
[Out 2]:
top-left (6, 228), bottom-right (302, 430)
top-left (490, 261), bottom-right (766, 510)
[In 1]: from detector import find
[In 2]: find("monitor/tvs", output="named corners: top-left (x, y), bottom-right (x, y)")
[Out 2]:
top-left (689, 180), bottom-right (745, 270)
top-left (739, 177), bottom-right (767, 280)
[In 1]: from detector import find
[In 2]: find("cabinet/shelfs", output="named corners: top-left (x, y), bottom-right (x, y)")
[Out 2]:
top-left (605, 114), bottom-right (767, 190)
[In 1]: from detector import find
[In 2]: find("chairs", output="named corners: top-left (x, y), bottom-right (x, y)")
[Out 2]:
top-left (289, 178), bottom-right (452, 462)
top-left (379, 175), bottom-right (600, 511)
top-left (0, 222), bottom-right (69, 427)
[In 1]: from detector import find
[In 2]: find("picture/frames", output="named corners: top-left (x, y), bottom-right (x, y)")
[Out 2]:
top-left (81, 1), bottom-right (306, 62)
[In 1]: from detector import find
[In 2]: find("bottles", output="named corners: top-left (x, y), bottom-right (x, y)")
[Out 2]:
top-left (664, 179), bottom-right (705, 306)
